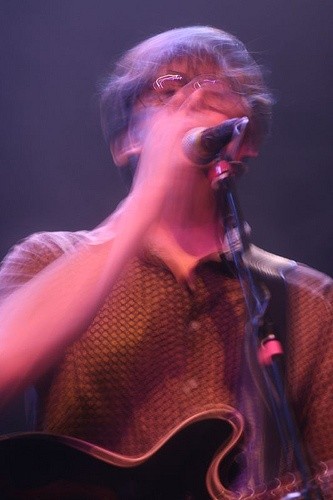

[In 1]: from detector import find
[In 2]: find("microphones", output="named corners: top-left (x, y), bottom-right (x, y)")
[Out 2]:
top-left (182, 115), bottom-right (248, 166)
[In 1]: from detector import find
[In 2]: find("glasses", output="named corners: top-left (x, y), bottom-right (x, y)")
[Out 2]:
top-left (138, 74), bottom-right (246, 106)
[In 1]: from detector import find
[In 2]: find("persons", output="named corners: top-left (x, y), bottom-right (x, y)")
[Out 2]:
top-left (0, 27), bottom-right (333, 500)
